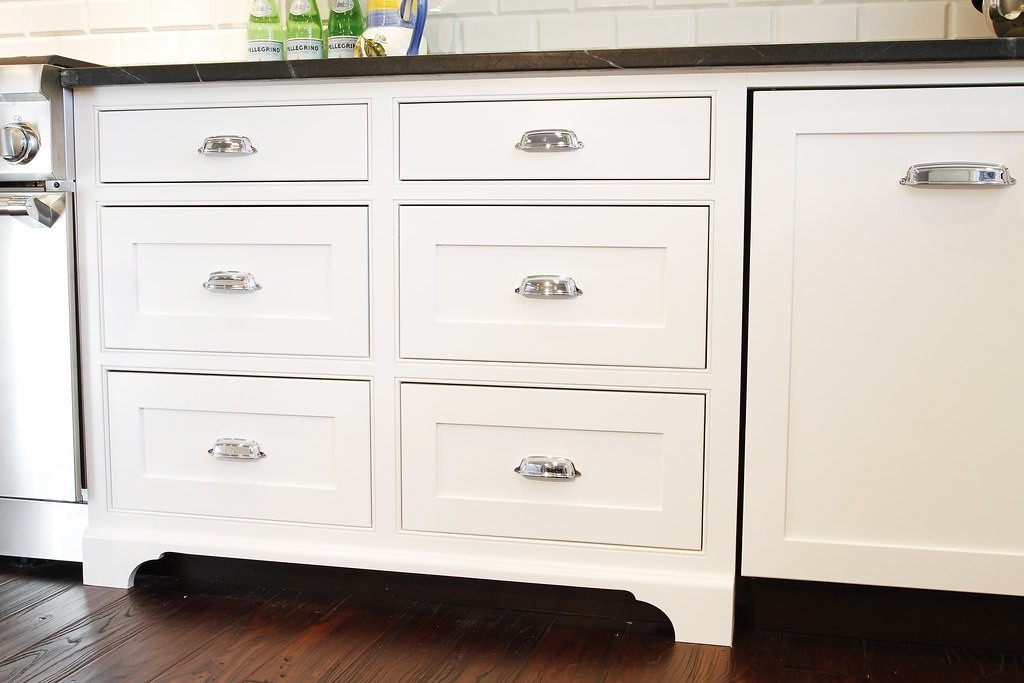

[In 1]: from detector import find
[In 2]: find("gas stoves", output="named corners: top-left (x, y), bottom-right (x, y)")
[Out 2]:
top-left (0, 52), bottom-right (107, 181)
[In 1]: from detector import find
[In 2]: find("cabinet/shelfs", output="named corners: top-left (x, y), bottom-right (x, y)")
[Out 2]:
top-left (66, 69), bottom-right (1024, 646)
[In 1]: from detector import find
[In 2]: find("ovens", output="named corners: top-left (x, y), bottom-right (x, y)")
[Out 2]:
top-left (0, 191), bottom-right (90, 502)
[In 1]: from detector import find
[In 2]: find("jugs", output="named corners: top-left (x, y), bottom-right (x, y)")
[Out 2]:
top-left (352, 0), bottom-right (428, 58)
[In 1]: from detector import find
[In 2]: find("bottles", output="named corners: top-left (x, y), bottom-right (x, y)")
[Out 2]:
top-left (247, 0), bottom-right (285, 62)
top-left (325, 0), bottom-right (365, 59)
top-left (283, 0), bottom-right (326, 61)
